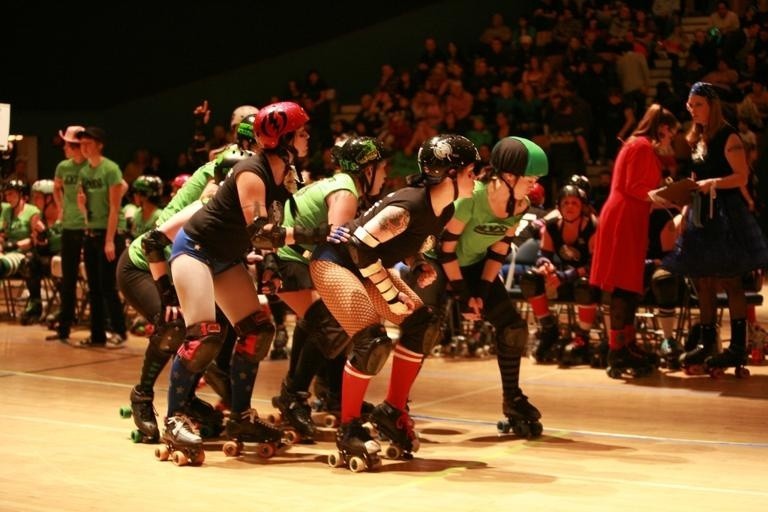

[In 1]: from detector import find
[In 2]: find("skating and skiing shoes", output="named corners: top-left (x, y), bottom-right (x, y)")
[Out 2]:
top-left (705, 348), bottom-right (750, 379)
top-left (268, 379), bottom-right (319, 444)
top-left (20, 300), bottom-right (42, 325)
top-left (46, 311), bottom-right (63, 330)
top-left (562, 333), bottom-right (590, 365)
top-left (659, 339), bottom-right (681, 368)
top-left (119, 387), bottom-right (159, 444)
top-left (461, 322), bottom-right (493, 357)
top-left (369, 402), bottom-right (420, 459)
top-left (223, 411), bottom-right (285, 458)
top-left (271, 329), bottom-right (288, 360)
top-left (679, 342), bottom-right (723, 374)
top-left (608, 342), bottom-right (660, 377)
top-left (192, 397), bottom-right (223, 440)
top-left (311, 380), bottom-right (376, 426)
top-left (155, 415), bottom-right (205, 465)
top-left (591, 340), bottom-right (608, 367)
top-left (328, 417), bottom-right (380, 472)
top-left (532, 322), bottom-right (570, 363)
top-left (497, 392), bottom-right (542, 437)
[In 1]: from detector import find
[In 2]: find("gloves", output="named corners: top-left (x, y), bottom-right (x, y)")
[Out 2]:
top-left (536, 256), bottom-right (551, 268)
top-left (1, 242), bottom-right (19, 252)
top-left (475, 280), bottom-right (494, 315)
top-left (38, 229), bottom-right (49, 241)
top-left (262, 254), bottom-right (284, 293)
top-left (153, 274), bottom-right (179, 306)
top-left (412, 263), bottom-right (423, 283)
top-left (556, 269), bottom-right (579, 286)
top-left (449, 279), bottom-right (473, 313)
top-left (294, 223), bottom-right (333, 243)
top-left (374, 277), bottom-right (408, 314)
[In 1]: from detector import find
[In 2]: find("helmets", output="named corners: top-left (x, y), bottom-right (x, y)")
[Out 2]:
top-left (557, 185), bottom-right (587, 208)
top-left (491, 137), bottom-right (549, 176)
top-left (418, 135), bottom-right (482, 185)
top-left (254, 102), bottom-right (309, 150)
top-left (237, 114), bottom-right (259, 144)
top-left (565, 174), bottom-right (591, 198)
top-left (339, 137), bottom-right (386, 171)
top-left (231, 106), bottom-right (260, 127)
top-left (171, 175), bottom-right (191, 186)
top-left (3, 178), bottom-right (28, 196)
top-left (31, 179), bottom-right (55, 194)
top-left (133, 175), bottom-right (163, 202)
top-left (214, 151), bottom-right (255, 185)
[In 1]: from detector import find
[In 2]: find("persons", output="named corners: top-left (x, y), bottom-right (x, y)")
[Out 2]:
top-left (520, 186), bottom-right (600, 360)
top-left (403, 135), bottom-right (550, 440)
top-left (273, 1), bottom-right (768, 159)
top-left (156, 102), bottom-right (311, 465)
top-left (74, 128), bottom-right (126, 347)
top-left (312, 131), bottom-right (482, 473)
top-left (192, 100), bottom-right (211, 140)
top-left (261, 136), bottom-right (395, 436)
top-left (20, 179), bottom-right (63, 324)
top-left (589, 104), bottom-right (682, 378)
top-left (155, 116), bottom-right (259, 418)
top-left (0, 176), bottom-right (40, 278)
top-left (47, 125), bottom-right (128, 341)
top-left (674, 81), bottom-right (762, 368)
top-left (121, 150), bottom-right (146, 184)
top-left (127, 175), bottom-right (161, 246)
top-left (114, 147), bottom-right (258, 441)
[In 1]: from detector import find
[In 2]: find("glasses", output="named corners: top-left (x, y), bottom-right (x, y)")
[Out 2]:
top-left (687, 102), bottom-right (706, 113)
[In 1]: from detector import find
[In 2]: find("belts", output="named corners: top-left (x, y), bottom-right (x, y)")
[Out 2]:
top-left (84, 229), bottom-right (106, 237)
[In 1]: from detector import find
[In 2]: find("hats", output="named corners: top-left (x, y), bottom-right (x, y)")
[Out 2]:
top-left (75, 126), bottom-right (104, 144)
top-left (59, 126), bottom-right (85, 144)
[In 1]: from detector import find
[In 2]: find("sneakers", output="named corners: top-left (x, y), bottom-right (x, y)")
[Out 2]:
top-left (73, 338), bottom-right (106, 348)
top-left (106, 335), bottom-right (127, 348)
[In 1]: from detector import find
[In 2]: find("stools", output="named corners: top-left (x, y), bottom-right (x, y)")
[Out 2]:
top-left (1, 259), bottom-right (129, 322)
